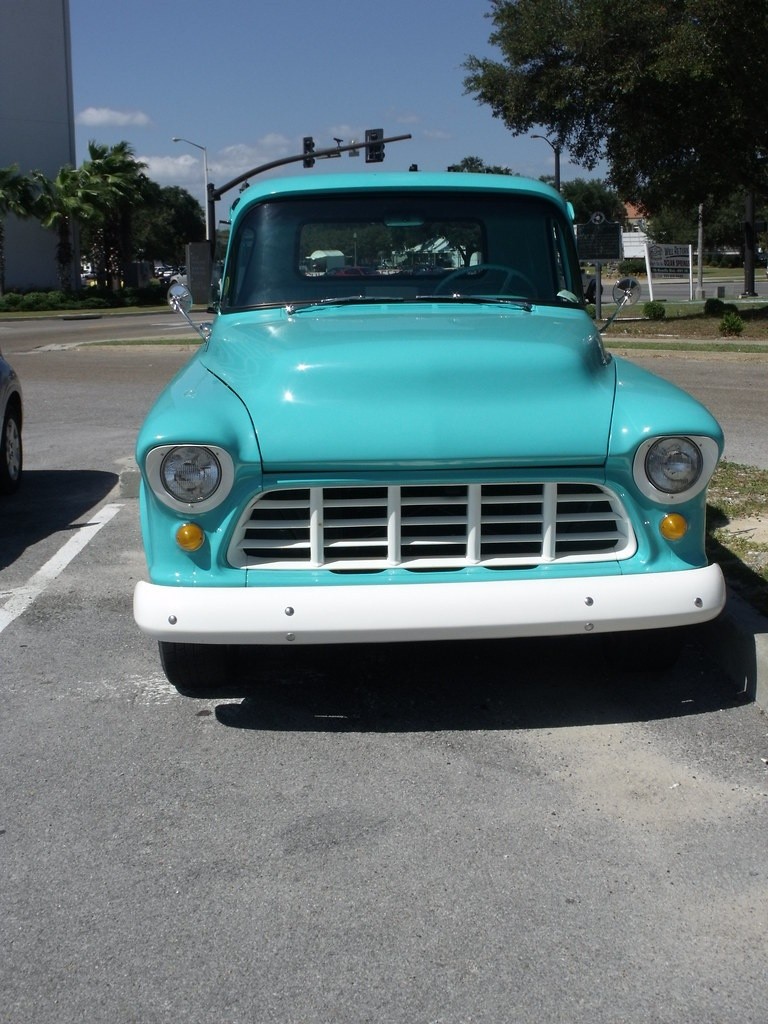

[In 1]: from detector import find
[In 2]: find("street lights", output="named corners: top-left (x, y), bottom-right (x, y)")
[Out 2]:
top-left (531, 134), bottom-right (560, 193)
top-left (352, 231), bottom-right (358, 267)
top-left (172, 137), bottom-right (209, 241)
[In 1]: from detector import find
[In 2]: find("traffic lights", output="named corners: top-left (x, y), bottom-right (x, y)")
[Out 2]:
top-left (303, 137), bottom-right (315, 168)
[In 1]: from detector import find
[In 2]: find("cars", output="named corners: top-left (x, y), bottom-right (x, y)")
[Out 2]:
top-left (0, 350), bottom-right (23, 495)
top-left (80, 258), bottom-right (188, 289)
top-left (320, 266), bottom-right (381, 276)
top-left (559, 262), bottom-right (603, 304)
top-left (133, 162), bottom-right (727, 692)
